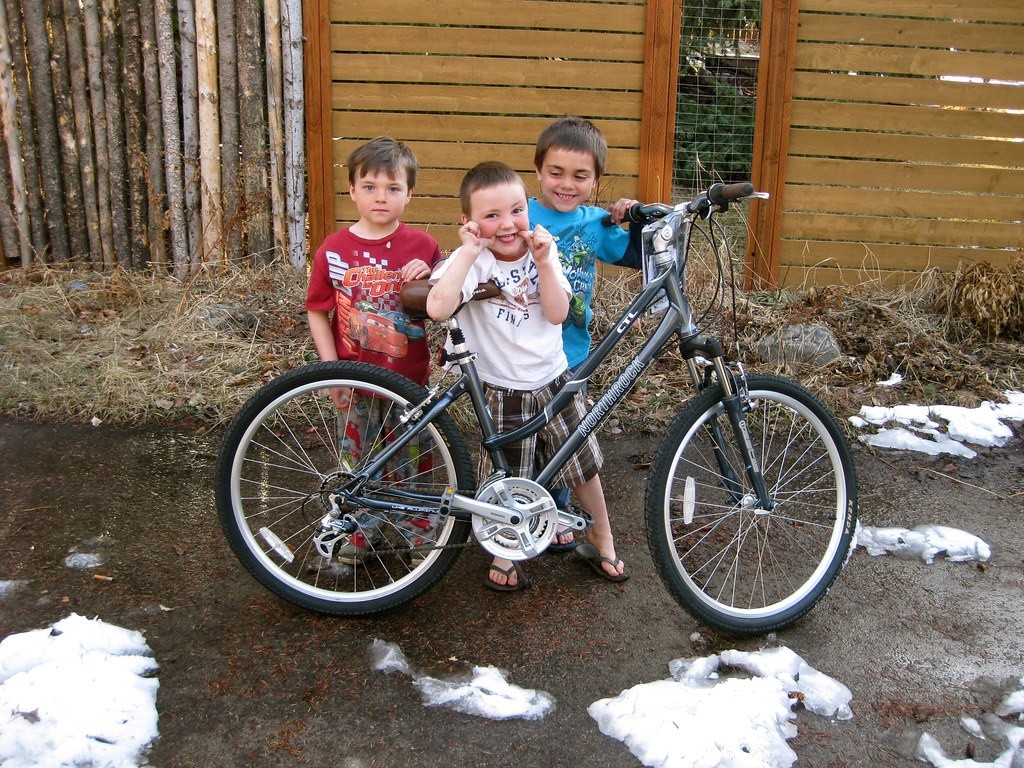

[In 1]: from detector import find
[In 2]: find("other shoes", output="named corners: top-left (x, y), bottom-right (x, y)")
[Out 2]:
top-left (337, 530), bottom-right (382, 564)
top-left (401, 528), bottom-right (437, 568)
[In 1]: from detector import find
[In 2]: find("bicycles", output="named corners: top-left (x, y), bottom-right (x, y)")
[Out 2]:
top-left (217, 181), bottom-right (860, 638)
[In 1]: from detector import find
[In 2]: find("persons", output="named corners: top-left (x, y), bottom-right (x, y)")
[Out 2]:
top-left (426, 161), bottom-right (631, 591)
top-left (430, 118), bottom-right (643, 551)
top-left (306, 137), bottom-right (443, 566)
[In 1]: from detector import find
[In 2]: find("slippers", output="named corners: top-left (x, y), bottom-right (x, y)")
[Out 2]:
top-left (546, 528), bottom-right (577, 551)
top-left (486, 564), bottom-right (520, 591)
top-left (575, 542), bottom-right (631, 582)
